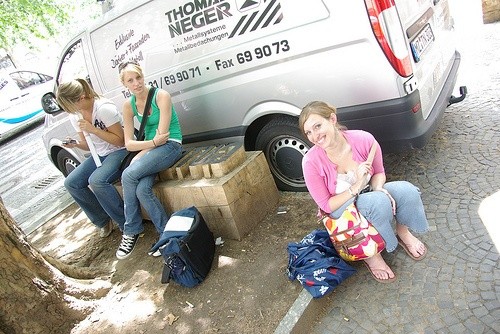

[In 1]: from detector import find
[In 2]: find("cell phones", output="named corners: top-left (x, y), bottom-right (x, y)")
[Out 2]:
top-left (360, 185), bottom-right (370, 194)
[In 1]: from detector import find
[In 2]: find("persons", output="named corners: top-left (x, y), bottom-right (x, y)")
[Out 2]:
top-left (115, 61), bottom-right (183, 260)
top-left (298, 102), bottom-right (430, 282)
top-left (55, 79), bottom-right (138, 238)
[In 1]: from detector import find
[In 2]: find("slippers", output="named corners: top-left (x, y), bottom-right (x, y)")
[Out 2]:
top-left (364, 258), bottom-right (396, 283)
top-left (398, 237), bottom-right (428, 259)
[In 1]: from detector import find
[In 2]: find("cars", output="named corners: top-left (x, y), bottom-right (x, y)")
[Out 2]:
top-left (0, 70), bottom-right (57, 146)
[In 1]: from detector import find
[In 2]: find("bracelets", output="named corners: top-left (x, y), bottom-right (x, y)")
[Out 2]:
top-left (348, 188), bottom-right (355, 197)
top-left (152, 141), bottom-right (156, 148)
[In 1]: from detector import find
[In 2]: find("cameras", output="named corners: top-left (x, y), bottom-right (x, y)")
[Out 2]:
top-left (62, 139), bottom-right (77, 145)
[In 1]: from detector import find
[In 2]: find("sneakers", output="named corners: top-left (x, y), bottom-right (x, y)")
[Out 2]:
top-left (152, 249), bottom-right (161, 257)
top-left (116, 228), bottom-right (138, 258)
top-left (100, 219), bottom-right (112, 237)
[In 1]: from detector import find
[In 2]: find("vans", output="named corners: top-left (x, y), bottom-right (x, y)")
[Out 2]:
top-left (43, 0), bottom-right (467, 192)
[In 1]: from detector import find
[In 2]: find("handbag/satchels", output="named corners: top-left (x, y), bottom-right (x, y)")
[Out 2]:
top-left (150, 206), bottom-right (217, 287)
top-left (118, 143), bottom-right (139, 170)
top-left (320, 206), bottom-right (385, 264)
